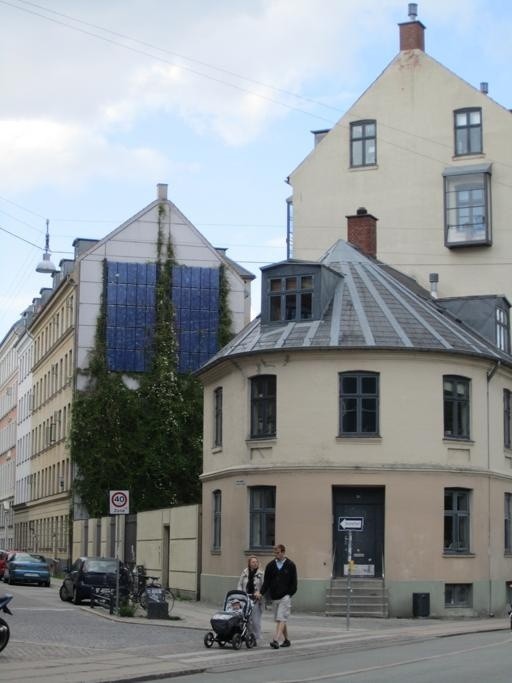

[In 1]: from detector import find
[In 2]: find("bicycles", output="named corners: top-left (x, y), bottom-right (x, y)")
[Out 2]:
top-left (98, 566), bottom-right (175, 613)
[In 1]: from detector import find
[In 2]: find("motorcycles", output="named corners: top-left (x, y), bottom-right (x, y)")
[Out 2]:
top-left (0, 592), bottom-right (14, 652)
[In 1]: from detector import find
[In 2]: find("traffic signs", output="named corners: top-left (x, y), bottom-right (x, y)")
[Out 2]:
top-left (110, 490), bottom-right (129, 514)
top-left (338, 515), bottom-right (363, 532)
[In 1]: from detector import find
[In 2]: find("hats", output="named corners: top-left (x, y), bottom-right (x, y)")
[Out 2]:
top-left (231, 599), bottom-right (239, 604)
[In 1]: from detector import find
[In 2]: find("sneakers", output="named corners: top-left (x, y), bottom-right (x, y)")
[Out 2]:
top-left (280, 640), bottom-right (290, 647)
top-left (270, 641), bottom-right (279, 648)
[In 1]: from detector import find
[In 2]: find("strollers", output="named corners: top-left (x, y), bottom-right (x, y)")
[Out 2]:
top-left (203, 589), bottom-right (259, 650)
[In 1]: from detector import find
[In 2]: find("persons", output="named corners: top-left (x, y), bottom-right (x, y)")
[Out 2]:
top-left (257, 544), bottom-right (298, 651)
top-left (228, 599), bottom-right (243, 616)
top-left (239, 555), bottom-right (266, 643)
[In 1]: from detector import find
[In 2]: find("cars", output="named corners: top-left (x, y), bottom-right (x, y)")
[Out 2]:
top-left (0, 550), bottom-right (50, 587)
top-left (59, 556), bottom-right (130, 604)
